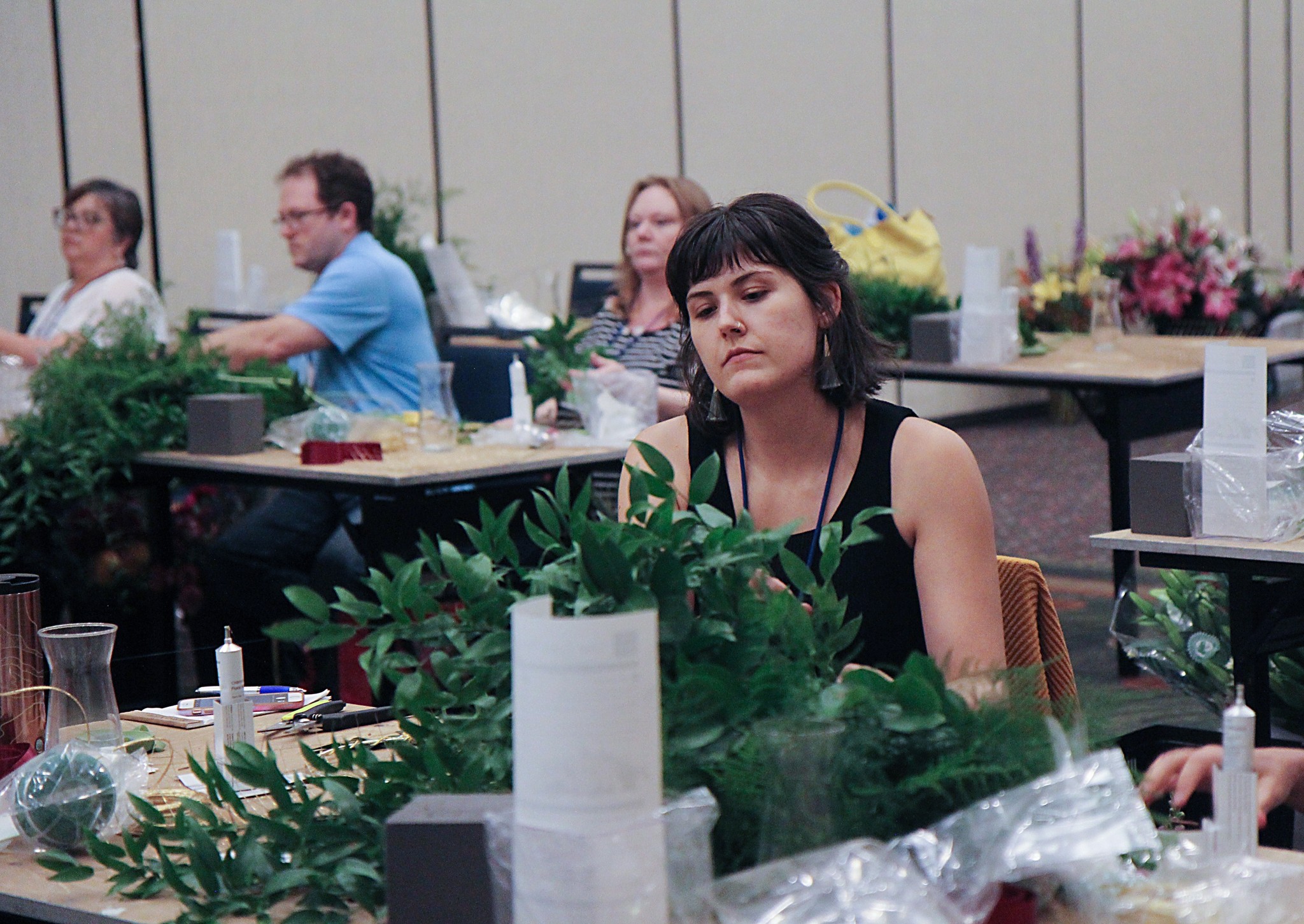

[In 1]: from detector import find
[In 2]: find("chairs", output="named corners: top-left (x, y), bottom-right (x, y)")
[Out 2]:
top-left (566, 262), bottom-right (620, 318)
top-left (437, 316), bottom-right (544, 419)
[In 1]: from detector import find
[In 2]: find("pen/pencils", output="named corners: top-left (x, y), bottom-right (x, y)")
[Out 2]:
top-left (194, 685), bottom-right (307, 694)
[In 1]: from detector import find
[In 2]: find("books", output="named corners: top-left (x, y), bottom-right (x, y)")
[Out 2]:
top-left (118, 688), bottom-right (331, 730)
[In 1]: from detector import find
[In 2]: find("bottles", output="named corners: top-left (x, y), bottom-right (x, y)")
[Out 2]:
top-left (1222, 684), bottom-right (1256, 771)
top-left (215, 626), bottom-right (245, 705)
top-left (508, 352), bottom-right (528, 397)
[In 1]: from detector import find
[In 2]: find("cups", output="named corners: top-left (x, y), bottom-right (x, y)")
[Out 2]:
top-left (0, 573), bottom-right (44, 752)
top-left (36, 623), bottom-right (125, 751)
top-left (416, 361), bottom-right (462, 450)
top-left (1089, 277), bottom-right (1124, 351)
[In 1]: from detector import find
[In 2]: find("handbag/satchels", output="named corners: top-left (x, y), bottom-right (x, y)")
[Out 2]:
top-left (809, 179), bottom-right (951, 295)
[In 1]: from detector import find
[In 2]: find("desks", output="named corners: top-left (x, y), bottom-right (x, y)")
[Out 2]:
top-left (1089, 530), bottom-right (1304, 746)
top-left (1, 700), bottom-right (1304, 924)
top-left (131, 430), bottom-right (631, 692)
top-left (882, 328), bottom-right (1304, 680)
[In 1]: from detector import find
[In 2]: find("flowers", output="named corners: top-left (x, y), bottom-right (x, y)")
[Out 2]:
top-left (1012, 196), bottom-right (1304, 338)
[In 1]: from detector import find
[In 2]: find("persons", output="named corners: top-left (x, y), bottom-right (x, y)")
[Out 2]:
top-left (0, 179), bottom-right (174, 588)
top-left (1137, 746), bottom-right (1304, 830)
top-left (176, 153), bottom-right (477, 696)
top-left (535, 176), bottom-right (714, 520)
top-left (617, 195), bottom-right (1008, 782)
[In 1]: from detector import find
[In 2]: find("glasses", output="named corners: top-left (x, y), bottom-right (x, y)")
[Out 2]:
top-left (51, 205), bottom-right (115, 233)
top-left (277, 200), bottom-right (344, 228)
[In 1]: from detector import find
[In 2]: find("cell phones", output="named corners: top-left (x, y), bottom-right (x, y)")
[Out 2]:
top-left (176, 690), bottom-right (305, 716)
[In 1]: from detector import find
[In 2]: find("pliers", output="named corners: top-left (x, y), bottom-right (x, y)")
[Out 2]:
top-left (257, 698), bottom-right (395, 734)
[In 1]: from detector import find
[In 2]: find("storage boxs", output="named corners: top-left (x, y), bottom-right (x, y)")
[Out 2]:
top-left (1129, 452), bottom-right (1192, 537)
top-left (386, 792), bottom-right (514, 924)
top-left (184, 390), bottom-right (264, 454)
top-left (1101, 724), bottom-right (1303, 850)
